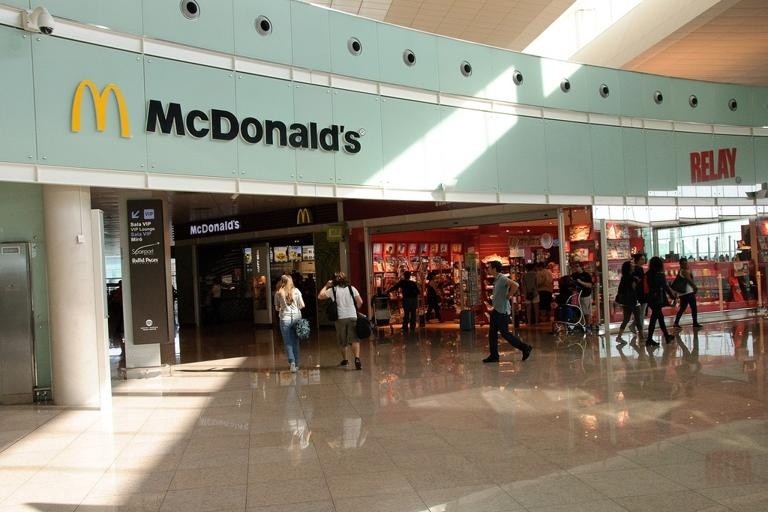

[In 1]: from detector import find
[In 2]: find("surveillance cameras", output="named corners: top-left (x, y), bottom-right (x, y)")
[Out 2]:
top-left (37, 12), bottom-right (56, 35)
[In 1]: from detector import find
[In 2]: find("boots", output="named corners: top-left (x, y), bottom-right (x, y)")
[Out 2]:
top-left (645, 338), bottom-right (660, 347)
top-left (665, 335), bottom-right (675, 344)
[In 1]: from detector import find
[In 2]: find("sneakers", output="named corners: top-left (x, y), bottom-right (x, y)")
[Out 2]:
top-left (355, 357), bottom-right (362, 369)
top-left (673, 323), bottom-right (683, 329)
top-left (639, 335), bottom-right (648, 342)
top-left (340, 360), bottom-right (348, 366)
top-left (616, 337), bottom-right (627, 344)
top-left (692, 323), bottom-right (703, 328)
top-left (521, 345), bottom-right (532, 361)
top-left (402, 326), bottom-right (415, 330)
top-left (289, 361), bottom-right (300, 373)
top-left (482, 354), bottom-right (499, 363)
top-left (629, 324), bottom-right (637, 334)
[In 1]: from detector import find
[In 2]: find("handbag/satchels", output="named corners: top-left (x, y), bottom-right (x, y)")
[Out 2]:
top-left (614, 286), bottom-right (637, 307)
top-left (670, 275), bottom-right (688, 294)
top-left (355, 312), bottom-right (371, 339)
top-left (326, 302), bottom-right (338, 322)
top-left (293, 318), bottom-right (310, 341)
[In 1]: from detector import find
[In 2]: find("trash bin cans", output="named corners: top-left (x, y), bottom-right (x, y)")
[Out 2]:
top-left (459, 310), bottom-right (472, 331)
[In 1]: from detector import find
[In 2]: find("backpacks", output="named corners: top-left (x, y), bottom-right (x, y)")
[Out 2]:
top-left (635, 273), bottom-right (655, 305)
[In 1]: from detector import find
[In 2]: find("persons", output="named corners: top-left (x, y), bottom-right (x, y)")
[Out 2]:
top-left (613, 253), bottom-right (703, 346)
top-left (679, 252), bottom-right (734, 262)
top-left (273, 274), bottom-right (306, 373)
top-left (205, 277), bottom-right (224, 326)
top-left (423, 271), bottom-right (443, 323)
top-left (521, 262), bottom-right (542, 327)
top-left (323, 366), bottom-right (379, 474)
top-left (316, 271), bottom-right (364, 371)
top-left (570, 261), bottom-right (595, 336)
top-left (481, 260), bottom-right (533, 363)
top-left (535, 260), bottom-right (555, 323)
top-left (616, 328), bottom-right (703, 418)
top-left (111, 279), bottom-right (124, 334)
top-left (385, 270), bottom-right (421, 334)
top-left (279, 371), bottom-right (315, 468)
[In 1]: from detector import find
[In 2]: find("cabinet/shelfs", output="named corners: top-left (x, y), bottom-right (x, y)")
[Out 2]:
top-left (480, 258), bottom-right (514, 323)
top-left (374, 242), bottom-right (466, 324)
top-left (604, 226), bottom-right (641, 322)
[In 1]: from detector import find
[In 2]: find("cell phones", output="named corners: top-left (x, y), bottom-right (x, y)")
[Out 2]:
top-left (332, 281), bottom-right (338, 286)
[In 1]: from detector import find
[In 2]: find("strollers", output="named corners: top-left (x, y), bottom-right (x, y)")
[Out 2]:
top-left (370, 293), bottom-right (395, 337)
top-left (550, 288), bottom-right (588, 340)
top-left (552, 341), bottom-right (588, 380)
